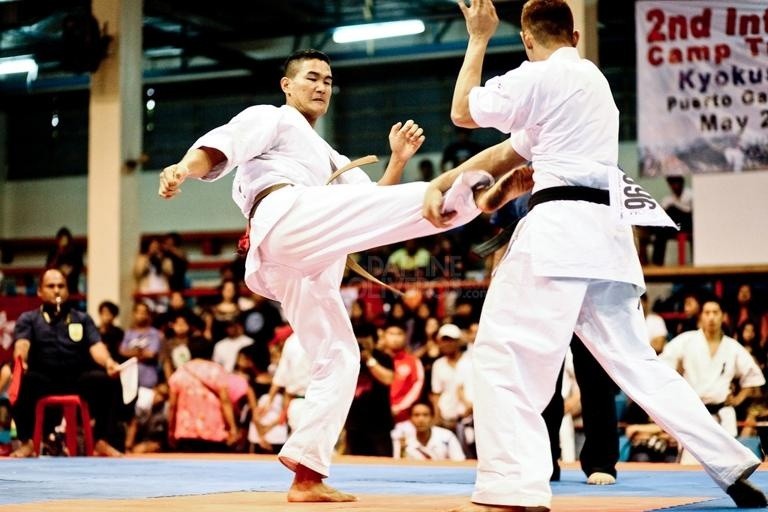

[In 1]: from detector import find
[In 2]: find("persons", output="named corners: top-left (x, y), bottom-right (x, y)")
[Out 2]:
top-left (1, 225), bottom-right (290, 459)
top-left (422, 1), bottom-right (768, 510)
top-left (562, 175), bottom-right (767, 467)
top-left (342, 127), bottom-right (495, 460)
top-left (158, 51), bottom-right (535, 503)
top-left (489, 154), bottom-right (620, 485)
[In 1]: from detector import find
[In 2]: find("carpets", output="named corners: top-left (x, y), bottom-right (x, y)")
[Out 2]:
top-left (0, 453), bottom-right (767, 510)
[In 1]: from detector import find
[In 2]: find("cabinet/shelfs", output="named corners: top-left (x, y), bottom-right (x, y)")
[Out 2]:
top-left (0, 228), bottom-right (247, 292)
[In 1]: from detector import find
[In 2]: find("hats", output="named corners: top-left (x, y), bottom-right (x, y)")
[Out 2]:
top-left (436, 322), bottom-right (461, 341)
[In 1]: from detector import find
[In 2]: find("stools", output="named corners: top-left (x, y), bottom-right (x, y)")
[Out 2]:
top-left (34, 395), bottom-right (97, 458)
